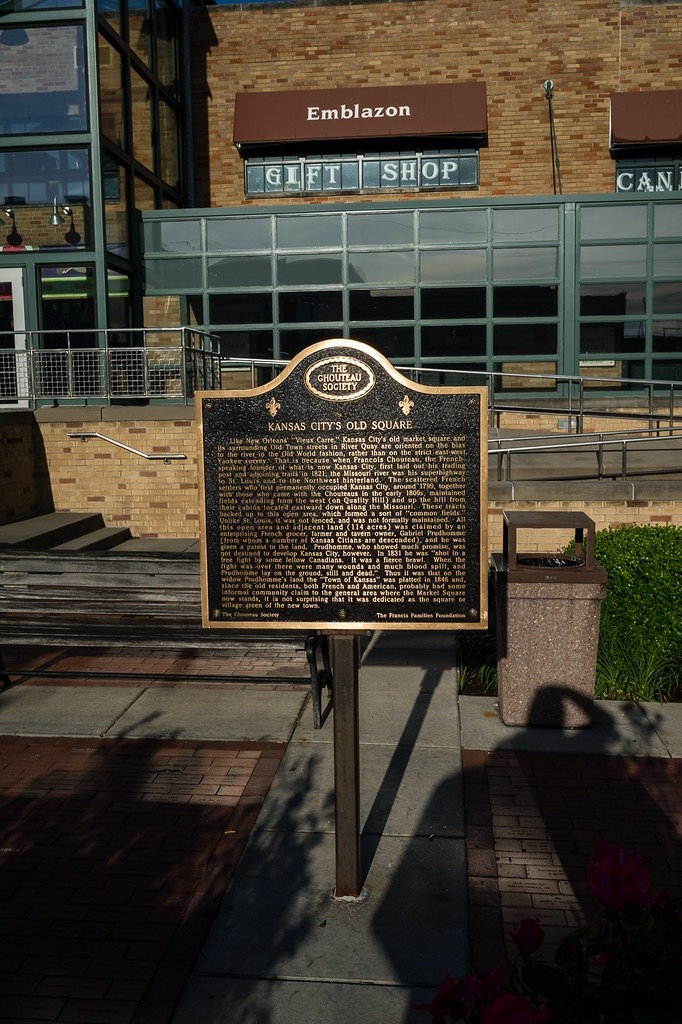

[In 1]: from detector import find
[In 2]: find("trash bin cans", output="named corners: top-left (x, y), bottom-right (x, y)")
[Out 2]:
top-left (490, 510), bottom-right (608, 727)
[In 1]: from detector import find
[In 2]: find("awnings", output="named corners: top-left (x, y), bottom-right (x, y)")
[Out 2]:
top-left (609, 90), bottom-right (681, 151)
top-left (232, 81), bottom-right (487, 150)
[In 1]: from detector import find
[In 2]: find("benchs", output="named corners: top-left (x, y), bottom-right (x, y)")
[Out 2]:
top-left (0, 544), bottom-right (336, 729)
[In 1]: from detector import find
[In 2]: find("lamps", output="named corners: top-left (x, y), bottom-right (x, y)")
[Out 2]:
top-left (48, 195), bottom-right (71, 226)
top-left (0, 204), bottom-right (13, 226)
